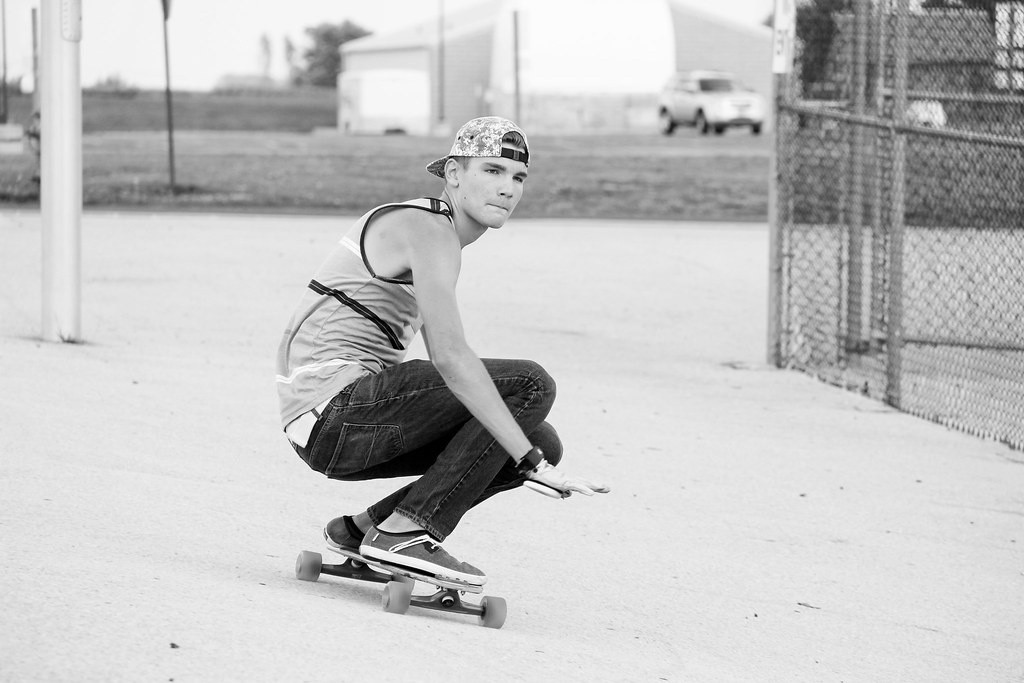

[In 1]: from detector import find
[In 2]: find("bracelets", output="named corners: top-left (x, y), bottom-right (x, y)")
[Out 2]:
top-left (513, 447), bottom-right (559, 483)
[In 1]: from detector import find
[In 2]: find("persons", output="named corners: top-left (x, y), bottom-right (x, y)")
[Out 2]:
top-left (274, 117), bottom-right (563, 585)
top-left (25, 115), bottom-right (40, 185)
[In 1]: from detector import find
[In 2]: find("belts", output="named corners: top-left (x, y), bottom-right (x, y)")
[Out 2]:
top-left (291, 393), bottom-right (337, 449)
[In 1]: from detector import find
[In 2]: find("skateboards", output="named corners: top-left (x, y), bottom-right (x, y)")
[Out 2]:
top-left (292, 542), bottom-right (509, 630)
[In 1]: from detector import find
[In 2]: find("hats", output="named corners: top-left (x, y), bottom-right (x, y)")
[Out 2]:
top-left (426, 116), bottom-right (531, 179)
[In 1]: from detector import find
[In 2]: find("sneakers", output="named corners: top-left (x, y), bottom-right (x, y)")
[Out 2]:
top-left (358, 524), bottom-right (488, 586)
top-left (324, 515), bottom-right (366, 555)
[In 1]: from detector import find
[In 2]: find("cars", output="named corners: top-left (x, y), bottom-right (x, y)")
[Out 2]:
top-left (656, 70), bottom-right (763, 138)
